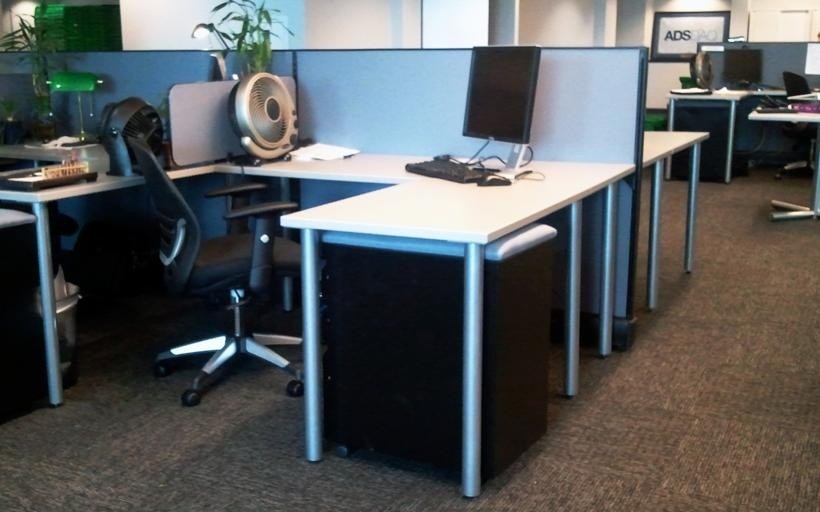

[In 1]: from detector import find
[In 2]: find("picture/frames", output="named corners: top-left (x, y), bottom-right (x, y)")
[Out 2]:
top-left (649, 10), bottom-right (729, 60)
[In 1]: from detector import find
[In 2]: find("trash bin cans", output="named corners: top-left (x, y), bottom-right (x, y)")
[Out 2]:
top-left (36, 279), bottom-right (80, 352)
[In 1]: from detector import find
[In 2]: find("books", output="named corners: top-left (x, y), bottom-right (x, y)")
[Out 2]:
top-left (787, 101), bottom-right (820, 113)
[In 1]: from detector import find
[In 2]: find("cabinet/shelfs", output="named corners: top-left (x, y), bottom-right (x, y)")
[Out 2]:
top-left (317, 222), bottom-right (557, 487)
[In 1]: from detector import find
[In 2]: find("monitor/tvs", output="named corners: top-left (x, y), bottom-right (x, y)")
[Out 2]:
top-left (462, 47), bottom-right (542, 181)
top-left (724, 49), bottom-right (762, 90)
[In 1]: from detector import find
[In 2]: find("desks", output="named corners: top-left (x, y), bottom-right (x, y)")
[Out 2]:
top-left (0, 147), bottom-right (217, 408)
top-left (280, 149), bottom-right (637, 499)
top-left (641, 124), bottom-right (713, 312)
top-left (747, 101), bottom-right (820, 223)
top-left (664, 86), bottom-right (786, 184)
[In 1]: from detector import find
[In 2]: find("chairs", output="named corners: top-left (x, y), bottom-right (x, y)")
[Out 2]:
top-left (98, 96), bottom-right (303, 406)
top-left (774, 68), bottom-right (817, 183)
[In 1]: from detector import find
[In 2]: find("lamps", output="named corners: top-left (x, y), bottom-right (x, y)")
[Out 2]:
top-left (45, 70), bottom-right (106, 148)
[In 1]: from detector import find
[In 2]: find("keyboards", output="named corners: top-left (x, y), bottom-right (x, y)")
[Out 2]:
top-left (406, 158), bottom-right (484, 183)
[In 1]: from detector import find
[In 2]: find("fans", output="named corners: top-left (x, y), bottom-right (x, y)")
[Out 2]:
top-left (228, 71), bottom-right (300, 164)
top-left (688, 50), bottom-right (715, 97)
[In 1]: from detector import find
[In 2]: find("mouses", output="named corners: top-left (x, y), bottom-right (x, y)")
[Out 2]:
top-left (479, 174), bottom-right (512, 186)
